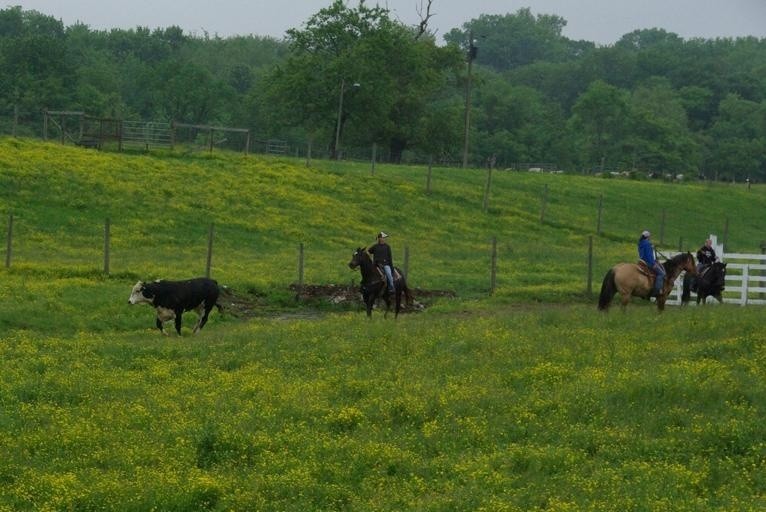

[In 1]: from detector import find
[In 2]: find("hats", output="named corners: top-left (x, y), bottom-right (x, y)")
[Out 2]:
top-left (378, 232), bottom-right (388, 238)
top-left (642, 231), bottom-right (651, 237)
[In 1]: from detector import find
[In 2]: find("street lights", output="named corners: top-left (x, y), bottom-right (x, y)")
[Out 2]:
top-left (461, 28), bottom-right (478, 168)
top-left (333, 79), bottom-right (361, 160)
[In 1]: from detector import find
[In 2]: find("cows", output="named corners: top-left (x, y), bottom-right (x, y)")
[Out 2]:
top-left (128, 277), bottom-right (224, 338)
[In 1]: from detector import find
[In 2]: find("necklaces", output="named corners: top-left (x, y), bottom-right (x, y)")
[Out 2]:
top-left (695, 238), bottom-right (719, 274)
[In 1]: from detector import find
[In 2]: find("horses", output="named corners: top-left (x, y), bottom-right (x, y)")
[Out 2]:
top-left (598, 251), bottom-right (699, 311)
top-left (681, 261), bottom-right (727, 305)
top-left (349, 246), bottom-right (409, 319)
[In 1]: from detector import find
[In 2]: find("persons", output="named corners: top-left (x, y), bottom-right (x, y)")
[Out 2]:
top-left (367, 232), bottom-right (396, 292)
top-left (638, 230), bottom-right (667, 295)
